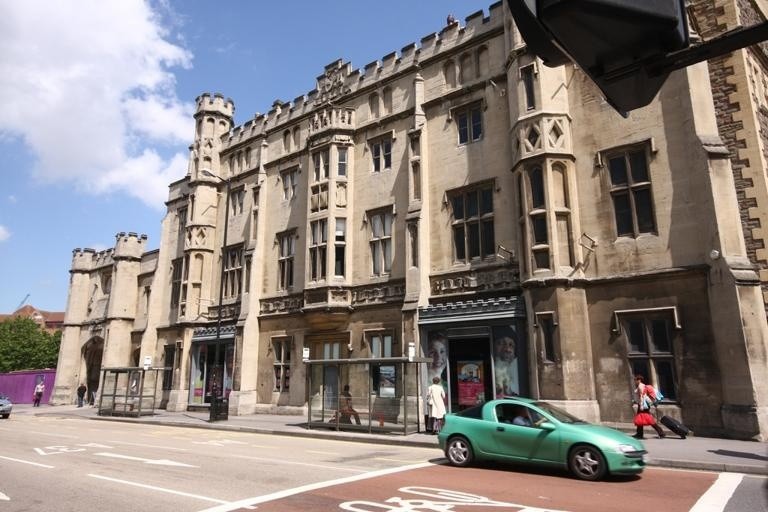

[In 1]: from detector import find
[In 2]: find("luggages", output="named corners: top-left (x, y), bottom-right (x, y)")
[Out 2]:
top-left (651, 408), bottom-right (688, 439)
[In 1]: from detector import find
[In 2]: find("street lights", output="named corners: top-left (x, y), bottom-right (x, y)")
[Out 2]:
top-left (199, 167), bottom-right (231, 423)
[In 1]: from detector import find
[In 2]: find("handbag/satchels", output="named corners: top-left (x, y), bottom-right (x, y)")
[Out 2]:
top-left (633, 411), bottom-right (656, 426)
top-left (654, 391), bottom-right (664, 405)
top-left (426, 393), bottom-right (433, 406)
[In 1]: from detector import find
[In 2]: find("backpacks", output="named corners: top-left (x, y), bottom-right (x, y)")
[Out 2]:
top-left (643, 384), bottom-right (655, 402)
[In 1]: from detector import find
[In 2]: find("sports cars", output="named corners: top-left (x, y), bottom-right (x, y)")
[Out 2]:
top-left (434, 394), bottom-right (648, 482)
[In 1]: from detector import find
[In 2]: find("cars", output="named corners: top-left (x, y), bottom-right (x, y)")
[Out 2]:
top-left (0, 393), bottom-right (13, 419)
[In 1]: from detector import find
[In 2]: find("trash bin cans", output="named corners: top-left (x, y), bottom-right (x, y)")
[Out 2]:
top-left (214, 397), bottom-right (228, 420)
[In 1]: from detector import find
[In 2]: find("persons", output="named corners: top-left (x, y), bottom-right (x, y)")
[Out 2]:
top-left (428, 329), bottom-right (448, 413)
top-left (493, 327), bottom-right (518, 395)
top-left (427, 377), bottom-right (446, 434)
top-left (339, 385), bottom-right (361, 424)
top-left (632, 375), bottom-right (666, 439)
top-left (32, 381), bottom-right (44, 407)
top-left (77, 383), bottom-right (86, 407)
top-left (513, 406), bottom-right (532, 427)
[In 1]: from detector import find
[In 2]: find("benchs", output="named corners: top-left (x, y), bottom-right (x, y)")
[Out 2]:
top-left (111, 402), bottom-right (135, 412)
